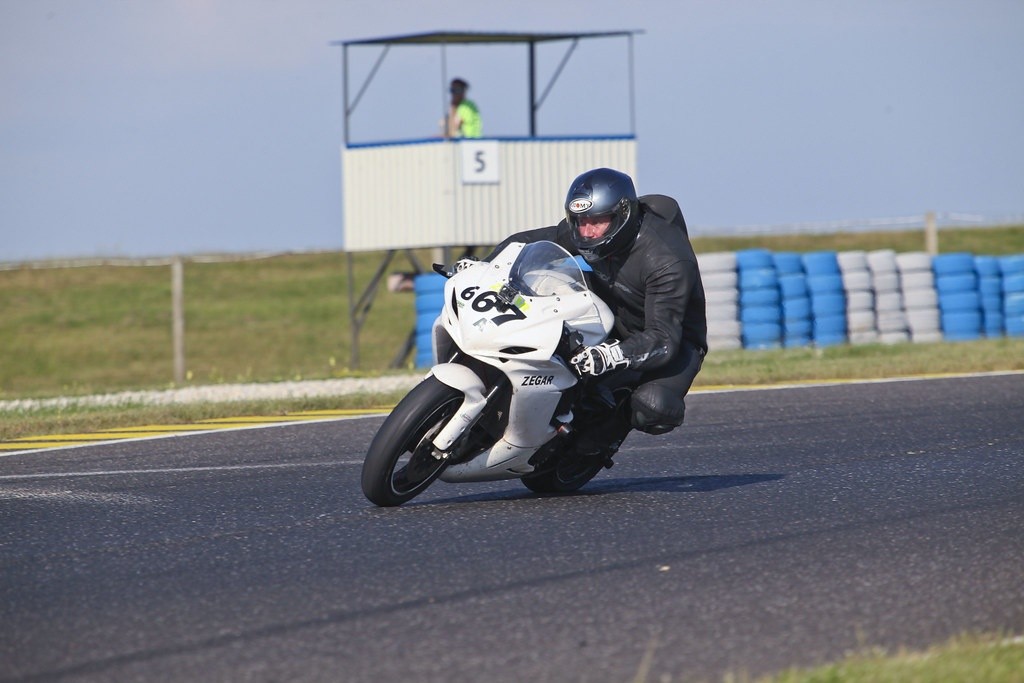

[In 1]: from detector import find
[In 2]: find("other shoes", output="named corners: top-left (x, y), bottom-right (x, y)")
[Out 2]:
top-left (574, 398), bottom-right (630, 456)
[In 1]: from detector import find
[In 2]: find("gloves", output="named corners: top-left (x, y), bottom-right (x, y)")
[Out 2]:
top-left (452, 256), bottom-right (481, 276)
top-left (569, 339), bottom-right (632, 376)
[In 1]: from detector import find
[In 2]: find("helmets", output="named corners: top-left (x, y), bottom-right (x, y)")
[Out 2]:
top-left (564, 169), bottom-right (639, 264)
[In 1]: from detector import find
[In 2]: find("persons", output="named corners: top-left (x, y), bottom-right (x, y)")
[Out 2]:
top-left (455, 168), bottom-right (708, 468)
top-left (442, 78), bottom-right (480, 139)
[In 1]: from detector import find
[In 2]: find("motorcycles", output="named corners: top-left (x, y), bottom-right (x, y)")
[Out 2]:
top-left (361, 240), bottom-right (633, 510)
